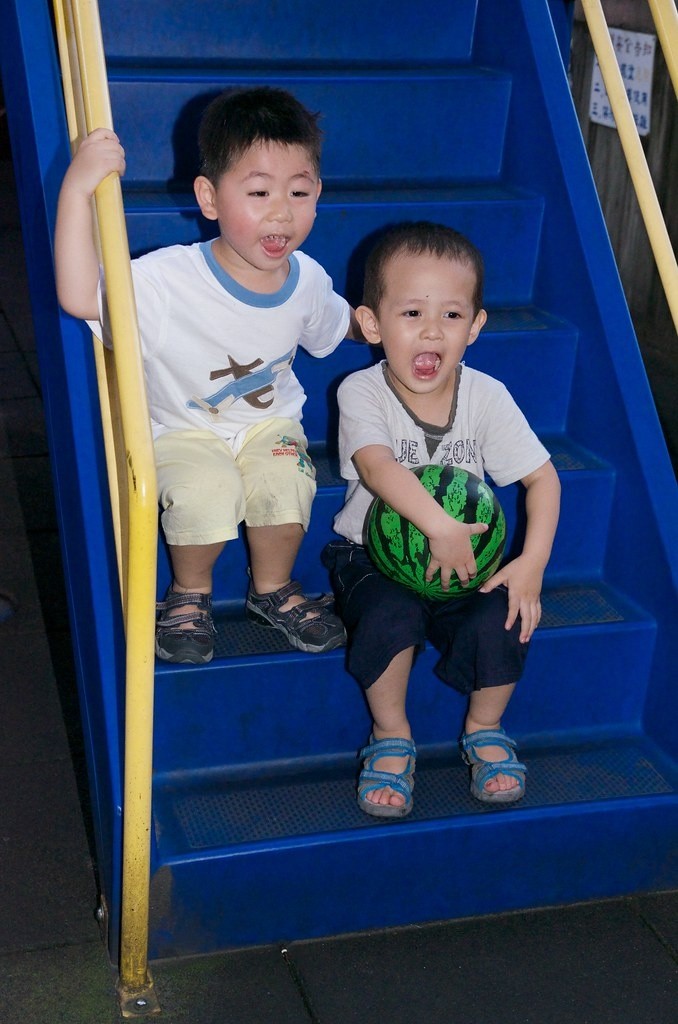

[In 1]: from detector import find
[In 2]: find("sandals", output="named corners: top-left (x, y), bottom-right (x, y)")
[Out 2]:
top-left (156, 585), bottom-right (219, 666)
top-left (461, 726), bottom-right (527, 802)
top-left (357, 733), bottom-right (417, 817)
top-left (244, 567), bottom-right (348, 653)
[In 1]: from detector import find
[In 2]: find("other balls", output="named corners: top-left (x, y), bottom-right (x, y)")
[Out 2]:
top-left (362, 464), bottom-right (507, 603)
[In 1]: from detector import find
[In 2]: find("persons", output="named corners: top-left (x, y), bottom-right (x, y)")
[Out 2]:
top-left (321, 221), bottom-right (561, 817)
top-left (55, 87), bottom-right (373, 665)
